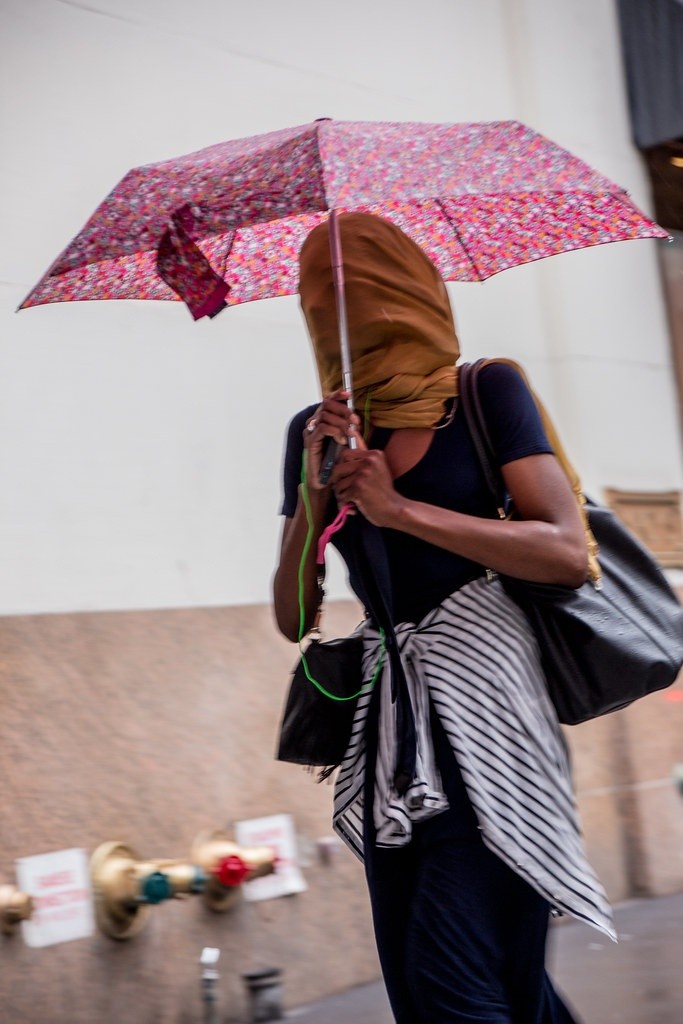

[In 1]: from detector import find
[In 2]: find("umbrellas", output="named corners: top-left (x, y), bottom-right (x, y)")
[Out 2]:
top-left (15, 119), bottom-right (675, 569)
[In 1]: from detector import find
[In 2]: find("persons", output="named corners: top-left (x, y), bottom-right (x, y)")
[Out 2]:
top-left (272, 211), bottom-right (592, 1024)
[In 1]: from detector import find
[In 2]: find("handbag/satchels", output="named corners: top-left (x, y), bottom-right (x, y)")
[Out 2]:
top-left (460, 358), bottom-right (683, 725)
top-left (277, 626), bottom-right (365, 784)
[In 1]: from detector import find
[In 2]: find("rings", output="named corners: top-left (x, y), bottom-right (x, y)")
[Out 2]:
top-left (306, 418), bottom-right (316, 432)
